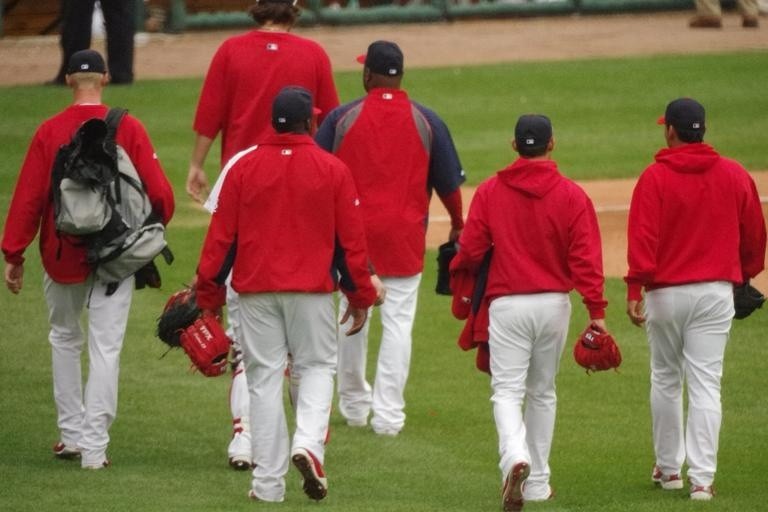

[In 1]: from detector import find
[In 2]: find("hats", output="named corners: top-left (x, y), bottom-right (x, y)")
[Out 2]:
top-left (354, 40), bottom-right (404, 77)
top-left (514, 114), bottom-right (552, 150)
top-left (271, 87), bottom-right (323, 124)
top-left (656, 97), bottom-right (705, 132)
top-left (62, 48), bottom-right (106, 74)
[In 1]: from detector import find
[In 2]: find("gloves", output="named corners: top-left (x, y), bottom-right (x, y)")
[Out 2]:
top-left (432, 243), bottom-right (460, 290)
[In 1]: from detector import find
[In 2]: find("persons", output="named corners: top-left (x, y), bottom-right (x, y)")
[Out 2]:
top-left (317, 40), bottom-right (465, 436)
top-left (45, 1), bottom-right (134, 85)
top-left (191, 86), bottom-right (376, 502)
top-left (202, 106), bottom-right (385, 469)
top-left (624, 98), bottom-right (766, 500)
top-left (2, 49), bottom-right (174, 468)
top-left (458, 114), bottom-right (619, 511)
top-left (689, 1), bottom-right (759, 30)
top-left (187, 1), bottom-right (339, 402)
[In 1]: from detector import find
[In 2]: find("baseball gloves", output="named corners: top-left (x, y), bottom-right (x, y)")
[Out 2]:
top-left (180, 315), bottom-right (232, 379)
top-left (573, 322), bottom-right (623, 372)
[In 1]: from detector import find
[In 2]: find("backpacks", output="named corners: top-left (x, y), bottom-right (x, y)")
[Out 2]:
top-left (49, 107), bottom-right (175, 296)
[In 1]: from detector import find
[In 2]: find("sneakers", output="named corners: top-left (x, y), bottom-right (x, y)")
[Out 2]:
top-left (247, 489), bottom-right (258, 500)
top-left (290, 447), bottom-right (328, 500)
top-left (501, 460), bottom-right (530, 512)
top-left (82, 458), bottom-right (112, 471)
top-left (651, 462), bottom-right (682, 490)
top-left (228, 456), bottom-right (251, 470)
top-left (687, 482), bottom-right (715, 503)
top-left (51, 441), bottom-right (80, 456)
